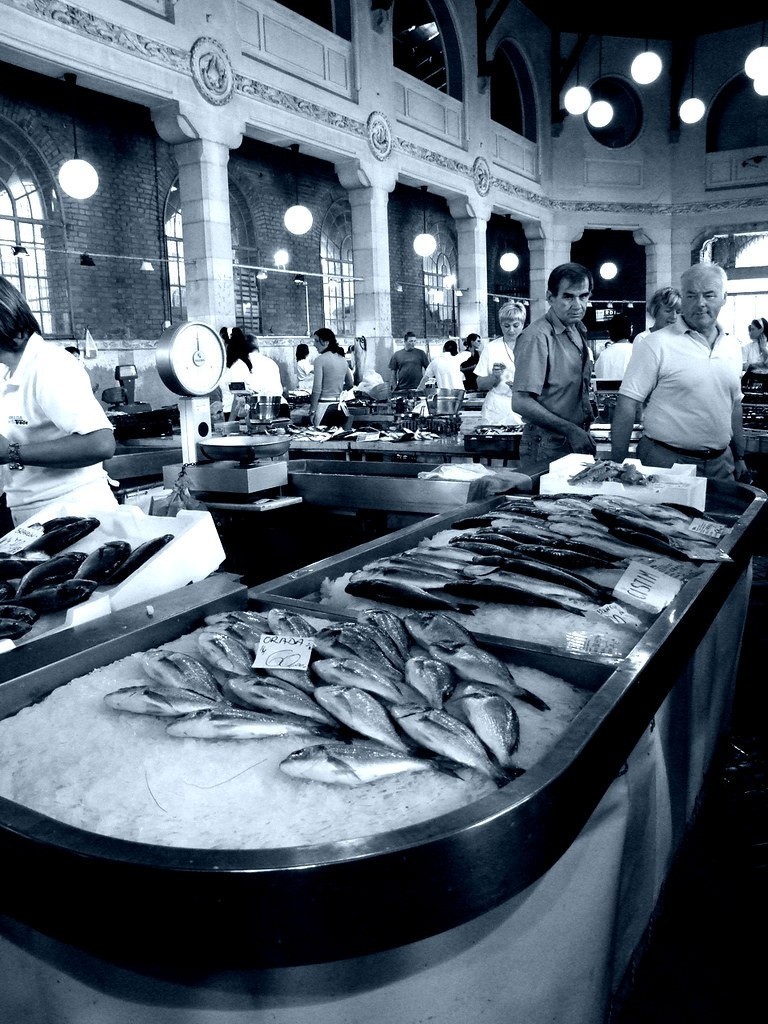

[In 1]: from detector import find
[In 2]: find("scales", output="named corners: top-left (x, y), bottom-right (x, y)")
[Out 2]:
top-left (101, 364), bottom-right (152, 413)
top-left (158, 322), bottom-right (291, 504)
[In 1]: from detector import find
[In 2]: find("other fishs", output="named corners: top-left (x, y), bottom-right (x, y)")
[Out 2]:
top-left (0, 514), bottom-right (176, 640)
top-left (102, 493), bottom-right (726, 791)
top-left (287, 425), bottom-right (440, 442)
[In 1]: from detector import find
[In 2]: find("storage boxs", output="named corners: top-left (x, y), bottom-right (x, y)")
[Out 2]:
top-left (539, 471), bottom-right (707, 512)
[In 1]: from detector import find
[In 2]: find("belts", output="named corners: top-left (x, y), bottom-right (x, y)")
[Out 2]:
top-left (656, 439), bottom-right (728, 459)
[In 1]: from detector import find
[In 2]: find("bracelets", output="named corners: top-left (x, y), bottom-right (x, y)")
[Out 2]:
top-left (736, 456), bottom-right (744, 460)
top-left (7, 442), bottom-right (24, 470)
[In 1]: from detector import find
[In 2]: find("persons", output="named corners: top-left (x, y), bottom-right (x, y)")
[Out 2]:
top-left (472, 300), bottom-right (525, 425)
top-left (295, 342), bottom-right (314, 392)
top-left (511, 261), bottom-right (597, 470)
top-left (416, 333), bottom-right (481, 411)
top-left (742, 317), bottom-right (768, 372)
top-left (312, 328), bottom-right (354, 427)
top-left (0, 277), bottom-right (116, 529)
top-left (610, 263), bottom-right (750, 484)
top-left (219, 325), bottom-right (291, 423)
top-left (595, 286), bottom-right (682, 381)
top-left (388, 331), bottom-right (430, 398)
top-left (66, 346), bottom-right (81, 360)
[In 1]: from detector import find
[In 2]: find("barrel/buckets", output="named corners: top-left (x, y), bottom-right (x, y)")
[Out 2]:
top-left (423, 388), bottom-right (465, 417)
top-left (246, 396), bottom-right (281, 420)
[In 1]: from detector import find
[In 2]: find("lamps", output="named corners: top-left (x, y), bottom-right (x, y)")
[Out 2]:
top-left (11, 246), bottom-right (30, 257)
top-left (140, 261), bottom-right (154, 271)
top-left (499, 214), bottom-right (519, 272)
top-left (284, 143), bottom-right (314, 235)
top-left (58, 72), bottom-right (100, 200)
top-left (79, 254), bottom-right (96, 267)
top-left (630, 5), bottom-right (663, 84)
top-left (413, 185), bottom-right (437, 257)
top-left (607, 303), bottom-right (613, 308)
top-left (393, 284), bottom-right (404, 293)
top-left (563, 16), bottom-right (592, 116)
top-left (454, 291), bottom-right (464, 297)
top-left (627, 303), bottom-right (633, 308)
top-left (256, 270), bottom-right (268, 279)
top-left (493, 296), bottom-right (500, 303)
top-left (293, 274), bottom-right (304, 284)
top-left (587, 12), bottom-right (614, 129)
top-left (599, 228), bottom-right (618, 280)
top-left (523, 299), bottom-right (530, 306)
top-left (743, 16), bottom-right (768, 96)
top-left (678, 48), bottom-right (706, 124)
